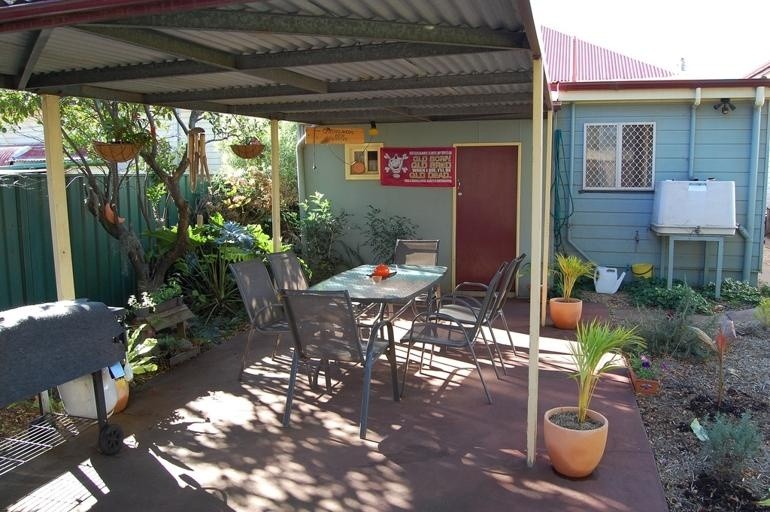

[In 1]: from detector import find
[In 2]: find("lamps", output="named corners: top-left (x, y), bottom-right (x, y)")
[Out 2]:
top-left (713, 98), bottom-right (736, 114)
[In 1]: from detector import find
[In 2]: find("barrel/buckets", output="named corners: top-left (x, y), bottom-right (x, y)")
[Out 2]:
top-left (630, 263), bottom-right (653, 280)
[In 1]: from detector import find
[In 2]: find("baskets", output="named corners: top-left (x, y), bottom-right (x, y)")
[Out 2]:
top-left (92, 139), bottom-right (143, 162)
top-left (230, 144), bottom-right (265, 159)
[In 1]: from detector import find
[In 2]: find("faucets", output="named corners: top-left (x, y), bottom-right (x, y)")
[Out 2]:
top-left (694, 225), bottom-right (701, 234)
top-left (634, 230), bottom-right (642, 242)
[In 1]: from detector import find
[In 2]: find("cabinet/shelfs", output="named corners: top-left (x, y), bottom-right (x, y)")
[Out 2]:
top-left (0, 299), bottom-right (127, 456)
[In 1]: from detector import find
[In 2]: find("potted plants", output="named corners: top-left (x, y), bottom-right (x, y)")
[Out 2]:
top-left (229, 136), bottom-right (267, 159)
top-left (549, 251), bottom-right (595, 330)
top-left (543, 314), bottom-right (649, 478)
top-left (90, 101), bottom-right (153, 162)
top-left (127, 281), bottom-right (184, 318)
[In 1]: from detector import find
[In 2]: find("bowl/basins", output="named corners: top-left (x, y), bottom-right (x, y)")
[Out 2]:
top-left (372, 276), bottom-right (382, 283)
